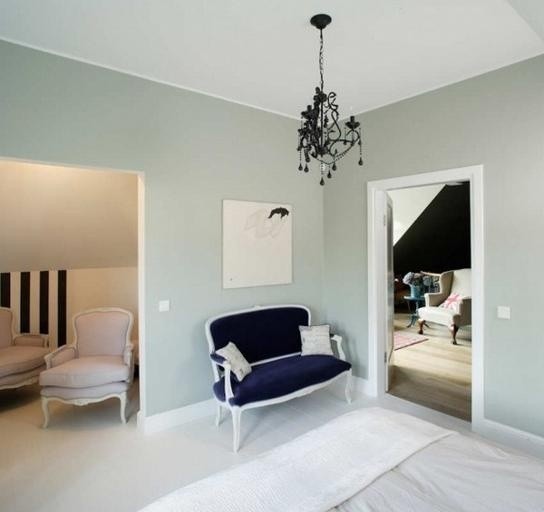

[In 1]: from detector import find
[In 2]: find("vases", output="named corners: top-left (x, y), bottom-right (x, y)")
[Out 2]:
top-left (411, 284), bottom-right (421, 298)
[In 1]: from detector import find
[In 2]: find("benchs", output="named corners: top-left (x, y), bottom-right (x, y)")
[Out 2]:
top-left (201, 302), bottom-right (355, 455)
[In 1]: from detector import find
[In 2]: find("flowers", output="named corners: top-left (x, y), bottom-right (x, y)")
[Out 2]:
top-left (401, 270), bottom-right (424, 288)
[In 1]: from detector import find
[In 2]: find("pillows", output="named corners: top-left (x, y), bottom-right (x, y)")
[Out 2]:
top-left (298, 322), bottom-right (337, 358)
top-left (438, 291), bottom-right (465, 312)
top-left (215, 340), bottom-right (253, 383)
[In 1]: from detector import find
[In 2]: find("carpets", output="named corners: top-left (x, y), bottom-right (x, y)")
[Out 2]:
top-left (392, 328), bottom-right (430, 352)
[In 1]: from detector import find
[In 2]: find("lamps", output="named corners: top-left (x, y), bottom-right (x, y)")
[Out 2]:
top-left (296, 12), bottom-right (365, 184)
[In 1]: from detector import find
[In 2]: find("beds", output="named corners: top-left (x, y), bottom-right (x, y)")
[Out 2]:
top-left (141, 404), bottom-right (543, 512)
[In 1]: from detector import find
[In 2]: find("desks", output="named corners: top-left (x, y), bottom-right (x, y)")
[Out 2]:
top-left (403, 295), bottom-right (430, 331)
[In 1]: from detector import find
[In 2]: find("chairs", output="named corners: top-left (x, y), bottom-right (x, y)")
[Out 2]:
top-left (39, 307), bottom-right (135, 429)
top-left (416, 268), bottom-right (472, 346)
top-left (0, 306), bottom-right (54, 391)
